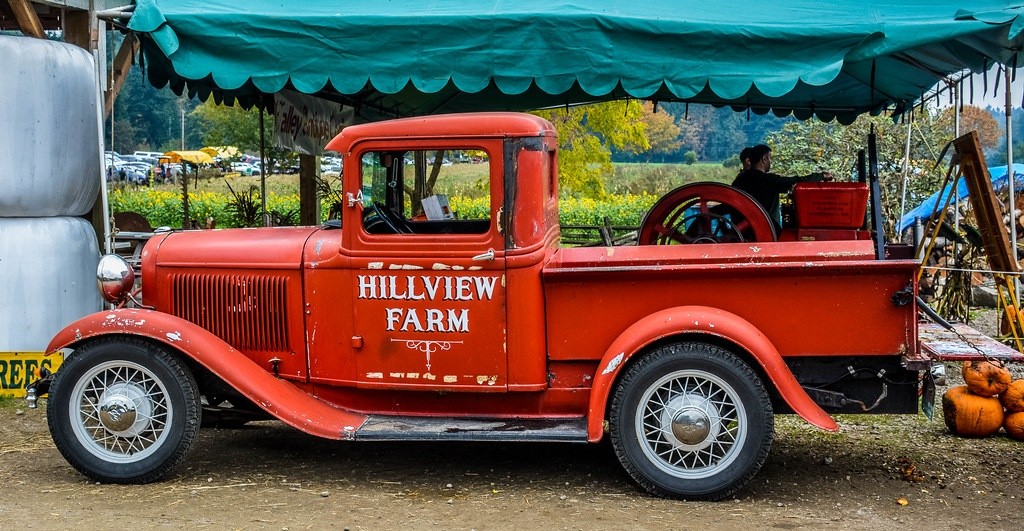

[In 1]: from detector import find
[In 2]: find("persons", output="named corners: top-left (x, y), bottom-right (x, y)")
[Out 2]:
top-left (131, 169), bottom-right (138, 186)
top-left (730, 144), bottom-right (834, 240)
top-left (117, 167), bottom-right (127, 181)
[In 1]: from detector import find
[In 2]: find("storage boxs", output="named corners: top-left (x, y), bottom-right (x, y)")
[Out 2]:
top-left (794, 183), bottom-right (870, 229)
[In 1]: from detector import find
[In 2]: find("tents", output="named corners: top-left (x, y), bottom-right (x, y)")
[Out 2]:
top-left (95, 1), bottom-right (1023, 317)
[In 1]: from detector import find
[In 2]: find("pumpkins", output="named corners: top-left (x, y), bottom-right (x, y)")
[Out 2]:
top-left (942, 360), bottom-right (1024, 438)
top-left (1001, 305), bottom-right (1024, 335)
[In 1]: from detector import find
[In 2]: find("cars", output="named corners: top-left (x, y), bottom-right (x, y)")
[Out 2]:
top-left (100, 145), bottom-right (490, 187)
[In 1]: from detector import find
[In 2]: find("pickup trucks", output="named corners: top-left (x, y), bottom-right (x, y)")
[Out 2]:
top-left (20, 108), bottom-right (941, 506)
